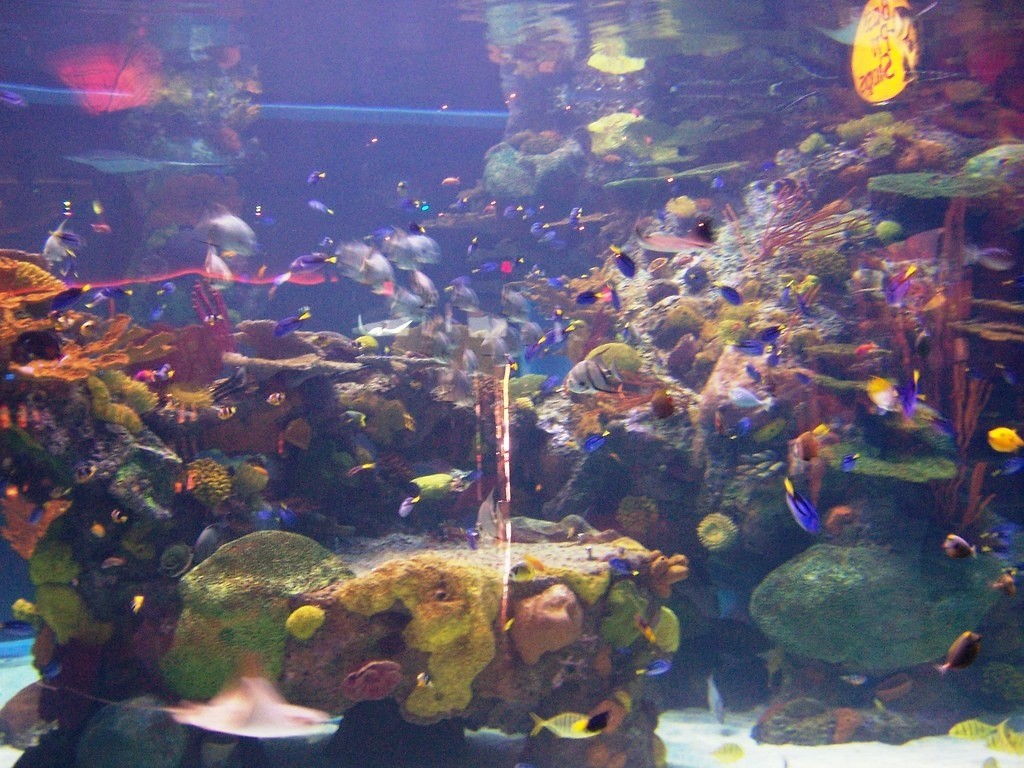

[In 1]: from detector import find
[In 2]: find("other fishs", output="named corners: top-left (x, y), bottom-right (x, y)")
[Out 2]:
top-left (0, 0), bottom-right (1023, 757)
